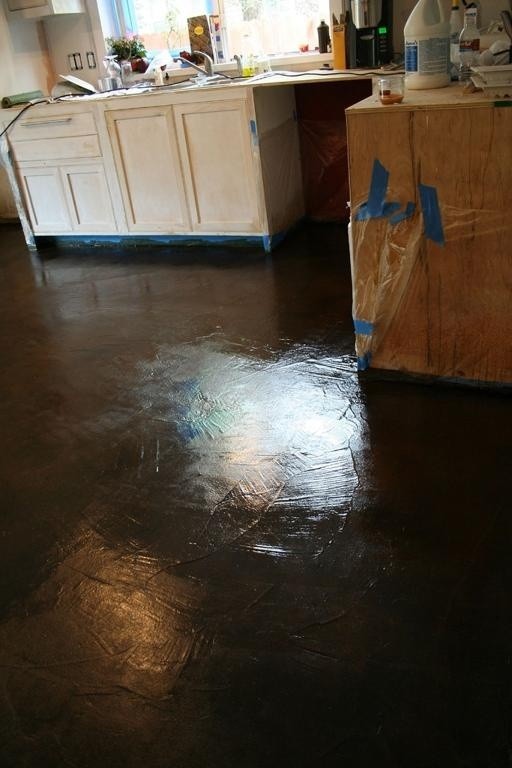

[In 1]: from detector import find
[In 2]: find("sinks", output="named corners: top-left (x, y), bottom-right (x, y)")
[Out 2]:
top-left (151, 83), bottom-right (194, 92)
top-left (205, 79), bottom-right (245, 85)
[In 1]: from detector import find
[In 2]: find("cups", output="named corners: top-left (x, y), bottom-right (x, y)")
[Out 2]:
top-left (377, 76), bottom-right (404, 106)
top-left (97, 77), bottom-right (113, 93)
top-left (120, 61), bottom-right (133, 88)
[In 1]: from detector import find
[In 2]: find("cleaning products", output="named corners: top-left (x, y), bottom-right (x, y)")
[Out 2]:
top-left (243, 34), bottom-right (255, 75)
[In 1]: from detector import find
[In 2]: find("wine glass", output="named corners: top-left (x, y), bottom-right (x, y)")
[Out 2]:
top-left (104, 54), bottom-right (122, 89)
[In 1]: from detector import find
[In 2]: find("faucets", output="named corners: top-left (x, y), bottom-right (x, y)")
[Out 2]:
top-left (233, 53), bottom-right (242, 76)
top-left (171, 55), bottom-right (206, 76)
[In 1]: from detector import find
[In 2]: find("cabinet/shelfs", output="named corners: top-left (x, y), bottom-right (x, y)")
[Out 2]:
top-left (0, 85), bottom-right (306, 256)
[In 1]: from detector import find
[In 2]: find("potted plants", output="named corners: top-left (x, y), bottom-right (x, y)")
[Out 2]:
top-left (106, 35), bottom-right (147, 72)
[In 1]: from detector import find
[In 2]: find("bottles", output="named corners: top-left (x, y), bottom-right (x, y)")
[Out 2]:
top-left (450, 2), bottom-right (463, 80)
top-left (456, 10), bottom-right (481, 85)
top-left (240, 33), bottom-right (254, 77)
top-left (317, 21), bottom-right (330, 53)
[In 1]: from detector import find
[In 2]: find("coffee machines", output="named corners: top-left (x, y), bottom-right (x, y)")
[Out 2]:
top-left (344, 0), bottom-right (395, 69)
